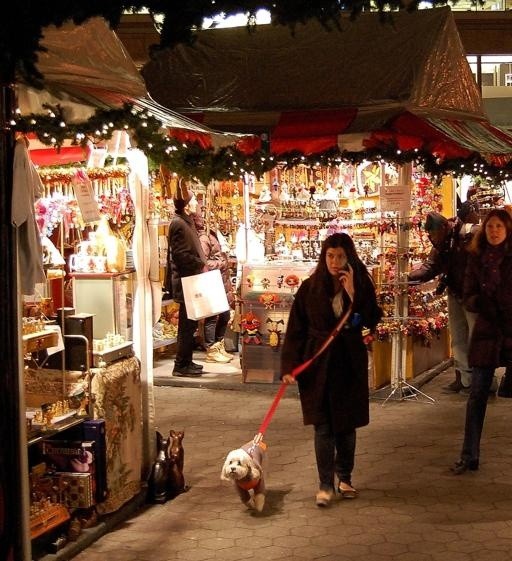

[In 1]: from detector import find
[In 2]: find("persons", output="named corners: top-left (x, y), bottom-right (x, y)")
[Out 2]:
top-left (446, 208), bottom-right (512, 477)
top-left (188, 204), bottom-right (235, 363)
top-left (406, 195), bottom-right (511, 403)
top-left (163, 189), bottom-right (213, 379)
top-left (279, 232), bottom-right (384, 507)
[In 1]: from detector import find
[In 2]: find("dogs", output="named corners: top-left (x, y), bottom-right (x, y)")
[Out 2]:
top-left (220, 441), bottom-right (268, 517)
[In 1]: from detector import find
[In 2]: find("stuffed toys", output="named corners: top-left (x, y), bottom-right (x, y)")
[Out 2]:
top-left (239, 161), bottom-right (451, 353)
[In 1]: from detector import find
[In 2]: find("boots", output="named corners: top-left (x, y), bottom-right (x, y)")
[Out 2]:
top-left (205, 342), bottom-right (231, 364)
top-left (216, 337), bottom-right (235, 360)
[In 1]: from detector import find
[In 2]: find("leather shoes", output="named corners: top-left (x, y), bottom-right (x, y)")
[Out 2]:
top-left (338, 480), bottom-right (359, 499)
top-left (449, 457), bottom-right (478, 472)
top-left (316, 484), bottom-right (336, 505)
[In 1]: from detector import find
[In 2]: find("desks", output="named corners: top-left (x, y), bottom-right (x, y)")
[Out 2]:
top-left (23, 356), bottom-right (143, 517)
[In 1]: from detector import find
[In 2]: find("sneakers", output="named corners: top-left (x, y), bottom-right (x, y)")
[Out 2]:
top-left (173, 364), bottom-right (203, 376)
top-left (450, 379), bottom-right (471, 391)
top-left (459, 387), bottom-right (473, 399)
top-left (188, 360), bottom-right (204, 370)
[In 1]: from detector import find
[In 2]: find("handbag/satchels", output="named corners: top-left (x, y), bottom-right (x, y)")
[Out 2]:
top-left (180, 267), bottom-right (231, 321)
top-left (163, 260), bottom-right (172, 290)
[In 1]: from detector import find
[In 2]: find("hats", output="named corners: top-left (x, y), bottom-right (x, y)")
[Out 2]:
top-left (424, 210), bottom-right (448, 230)
top-left (173, 189), bottom-right (194, 209)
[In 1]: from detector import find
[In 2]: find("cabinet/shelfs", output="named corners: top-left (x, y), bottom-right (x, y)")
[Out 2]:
top-left (20, 316), bottom-right (93, 547)
top-left (69, 265), bottom-right (137, 342)
top-left (141, 214), bottom-right (200, 351)
